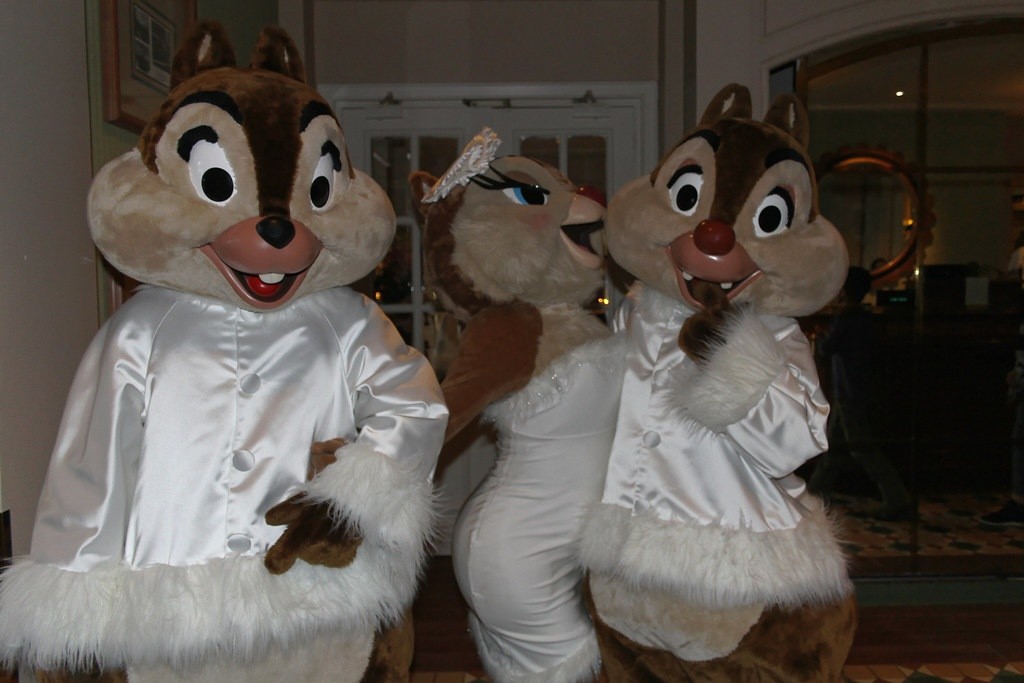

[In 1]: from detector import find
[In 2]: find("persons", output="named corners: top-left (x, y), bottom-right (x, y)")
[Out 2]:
top-left (830, 272), bottom-right (881, 361)
top-left (871, 257), bottom-right (896, 300)
top-left (976, 246), bottom-right (1024, 526)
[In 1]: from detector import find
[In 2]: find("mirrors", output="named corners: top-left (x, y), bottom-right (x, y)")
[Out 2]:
top-left (812, 141), bottom-right (935, 288)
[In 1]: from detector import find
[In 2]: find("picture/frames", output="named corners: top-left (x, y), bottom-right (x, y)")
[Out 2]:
top-left (99, 0), bottom-right (198, 135)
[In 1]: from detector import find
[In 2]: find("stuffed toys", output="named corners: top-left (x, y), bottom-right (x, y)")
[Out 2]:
top-left (410, 127), bottom-right (627, 676)
top-left (588, 76), bottom-right (891, 682)
top-left (1, 24), bottom-right (450, 683)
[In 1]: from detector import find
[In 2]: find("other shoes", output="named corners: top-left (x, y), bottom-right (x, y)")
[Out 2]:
top-left (980, 500), bottom-right (1023, 526)
top-left (865, 496), bottom-right (918, 520)
